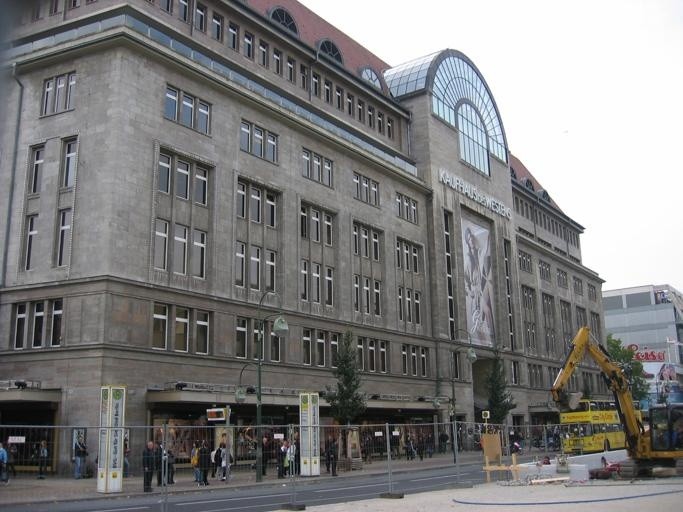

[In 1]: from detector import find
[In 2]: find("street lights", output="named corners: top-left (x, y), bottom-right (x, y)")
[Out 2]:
top-left (433, 328), bottom-right (478, 463)
top-left (234, 289), bottom-right (289, 483)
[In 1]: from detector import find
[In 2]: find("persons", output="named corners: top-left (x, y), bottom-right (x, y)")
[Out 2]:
top-left (360, 431), bottom-right (449, 464)
top-left (553, 425), bottom-right (560, 451)
top-left (220, 433), bottom-right (228, 446)
top-left (517, 427), bottom-right (526, 454)
top-left (0, 440), bottom-right (49, 485)
top-left (124, 437), bottom-right (131, 477)
top-left (457, 426), bottom-right (464, 453)
top-left (463, 226), bottom-right (485, 302)
top-left (510, 427), bottom-right (517, 448)
top-left (262, 431), bottom-right (300, 477)
top-left (325, 435), bottom-right (338, 476)
top-left (75, 436), bottom-right (88, 479)
top-left (190, 440), bottom-right (233, 485)
top-left (143, 441), bottom-right (176, 492)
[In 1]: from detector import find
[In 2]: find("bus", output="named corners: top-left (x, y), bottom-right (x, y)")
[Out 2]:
top-left (559, 399), bottom-right (643, 456)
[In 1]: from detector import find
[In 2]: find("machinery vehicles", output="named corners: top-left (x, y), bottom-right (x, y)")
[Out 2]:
top-left (550, 325), bottom-right (683, 479)
